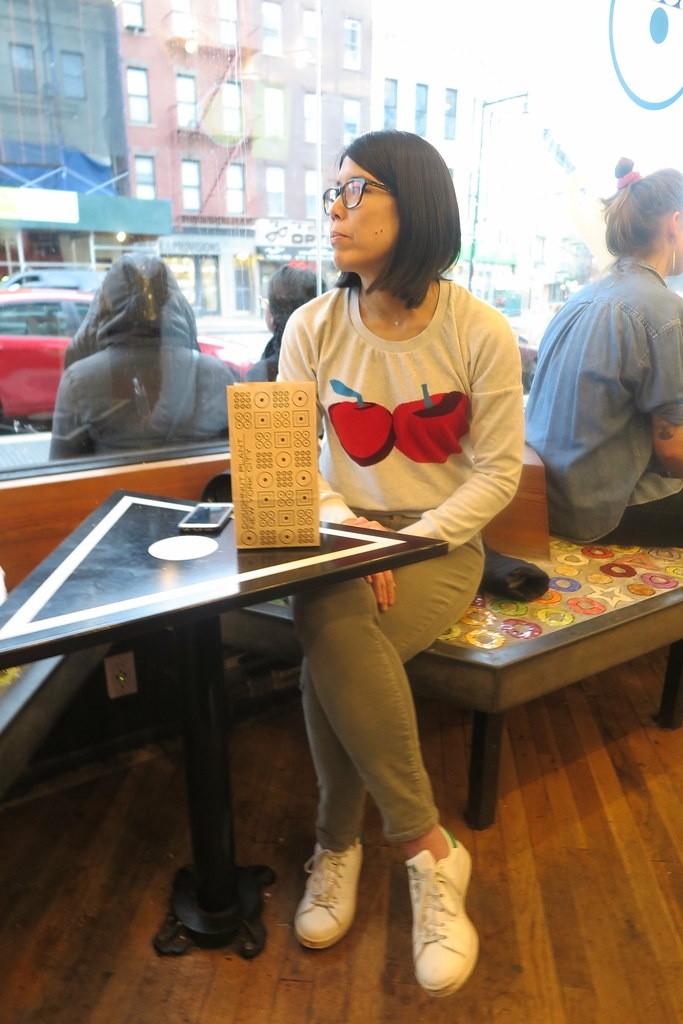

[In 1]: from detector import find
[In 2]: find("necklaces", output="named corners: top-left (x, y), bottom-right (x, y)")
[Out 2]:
top-left (363, 291), bottom-right (430, 326)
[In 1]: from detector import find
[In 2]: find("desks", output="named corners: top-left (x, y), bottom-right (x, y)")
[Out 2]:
top-left (0, 488), bottom-right (450, 963)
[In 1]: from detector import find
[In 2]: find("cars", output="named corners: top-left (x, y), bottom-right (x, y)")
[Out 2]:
top-left (0, 267), bottom-right (106, 333)
top-left (0, 283), bottom-right (258, 433)
top-left (513, 327), bottom-right (539, 390)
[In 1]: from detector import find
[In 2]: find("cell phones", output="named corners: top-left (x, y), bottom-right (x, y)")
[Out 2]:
top-left (176, 501), bottom-right (233, 534)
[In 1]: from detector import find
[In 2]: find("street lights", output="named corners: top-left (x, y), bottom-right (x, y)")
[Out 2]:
top-left (468, 92), bottom-right (529, 289)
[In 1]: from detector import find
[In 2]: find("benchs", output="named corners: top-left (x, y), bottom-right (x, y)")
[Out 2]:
top-left (217, 530), bottom-right (683, 831)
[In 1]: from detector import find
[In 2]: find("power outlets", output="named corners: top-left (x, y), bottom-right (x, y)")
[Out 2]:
top-left (104, 651), bottom-right (139, 699)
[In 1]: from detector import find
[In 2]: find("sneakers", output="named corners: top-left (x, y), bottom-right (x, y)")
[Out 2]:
top-left (294, 831), bottom-right (363, 949)
top-left (405, 825), bottom-right (480, 998)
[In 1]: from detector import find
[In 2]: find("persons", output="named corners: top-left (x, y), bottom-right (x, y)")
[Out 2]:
top-left (49, 252), bottom-right (236, 461)
top-left (276, 129), bottom-right (524, 997)
top-left (246, 264), bottom-right (329, 383)
top-left (523, 157), bottom-right (683, 548)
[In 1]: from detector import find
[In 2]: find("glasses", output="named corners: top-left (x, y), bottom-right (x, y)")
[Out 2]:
top-left (259, 294), bottom-right (270, 310)
top-left (322, 178), bottom-right (393, 215)
